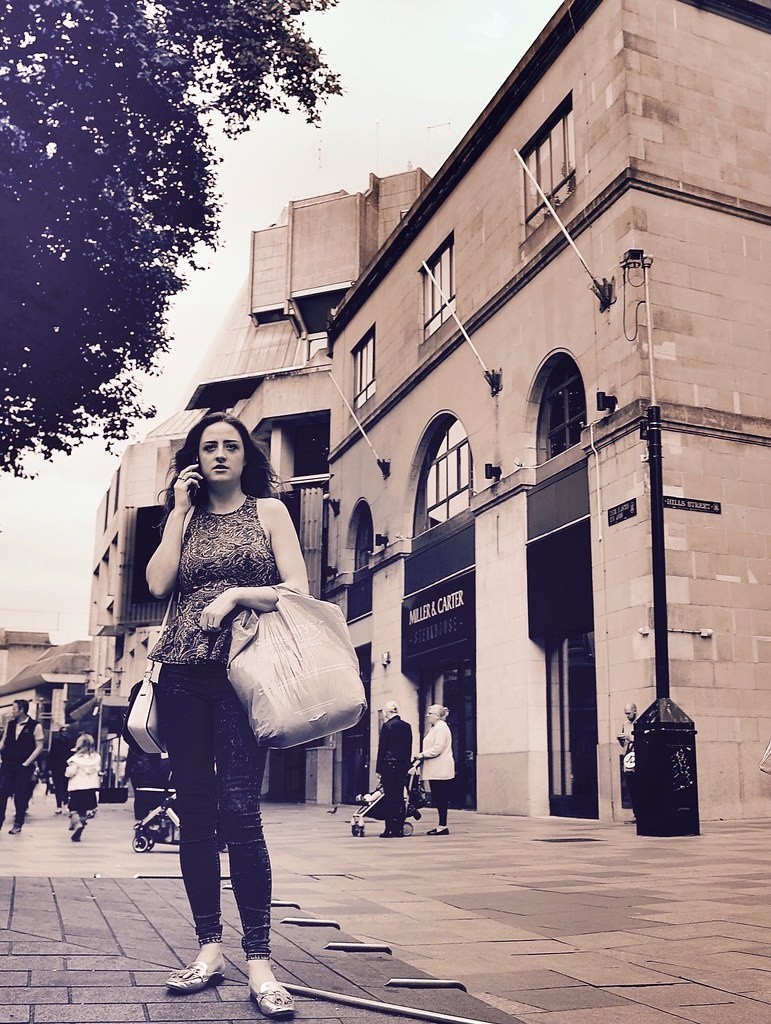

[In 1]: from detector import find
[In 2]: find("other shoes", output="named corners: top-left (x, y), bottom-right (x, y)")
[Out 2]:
top-left (9, 827), bottom-right (22, 834)
top-left (55, 808), bottom-right (62, 814)
top-left (164, 962), bottom-right (226, 995)
top-left (379, 828), bottom-right (403, 837)
top-left (427, 828), bottom-right (449, 835)
top-left (248, 975), bottom-right (296, 1019)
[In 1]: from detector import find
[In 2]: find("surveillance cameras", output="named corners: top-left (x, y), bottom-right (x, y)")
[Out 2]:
top-left (619, 249), bottom-right (644, 268)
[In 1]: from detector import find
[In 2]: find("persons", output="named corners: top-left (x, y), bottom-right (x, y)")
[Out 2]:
top-left (356, 785), bottom-right (384, 801)
top-left (46, 727), bottom-right (73, 813)
top-left (146, 412), bottom-right (307, 1018)
top-left (0, 699), bottom-right (45, 835)
top-left (374, 702), bottom-right (413, 838)
top-left (120, 746), bottom-right (155, 821)
top-left (414, 705), bottom-right (455, 835)
top-left (64, 734), bottom-right (101, 840)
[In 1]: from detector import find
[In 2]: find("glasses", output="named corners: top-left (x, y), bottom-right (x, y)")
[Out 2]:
top-left (429, 712), bottom-right (438, 715)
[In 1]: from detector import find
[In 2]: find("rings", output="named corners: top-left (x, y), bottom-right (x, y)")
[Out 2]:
top-left (177, 476), bottom-right (182, 479)
top-left (207, 623), bottom-right (213, 628)
top-left (181, 478), bottom-right (187, 481)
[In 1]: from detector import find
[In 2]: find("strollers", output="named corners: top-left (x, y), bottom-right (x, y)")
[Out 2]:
top-left (131, 765), bottom-right (183, 852)
top-left (352, 758), bottom-right (422, 836)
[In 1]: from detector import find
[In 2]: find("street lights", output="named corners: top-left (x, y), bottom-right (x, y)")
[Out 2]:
top-left (622, 249), bottom-right (699, 836)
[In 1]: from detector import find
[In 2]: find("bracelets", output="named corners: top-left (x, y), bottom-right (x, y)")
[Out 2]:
top-left (421, 753), bottom-right (424, 758)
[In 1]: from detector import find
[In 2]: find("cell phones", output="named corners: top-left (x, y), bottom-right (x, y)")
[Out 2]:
top-left (191, 456), bottom-right (199, 498)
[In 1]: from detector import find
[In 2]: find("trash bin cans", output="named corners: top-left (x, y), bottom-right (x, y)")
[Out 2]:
top-left (632, 697), bottom-right (701, 838)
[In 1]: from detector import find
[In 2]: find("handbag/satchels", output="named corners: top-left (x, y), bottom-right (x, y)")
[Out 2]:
top-left (226, 582), bottom-right (367, 749)
top-left (123, 680), bottom-right (168, 754)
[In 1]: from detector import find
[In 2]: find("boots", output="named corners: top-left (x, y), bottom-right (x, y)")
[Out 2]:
top-left (69, 814), bottom-right (84, 842)
